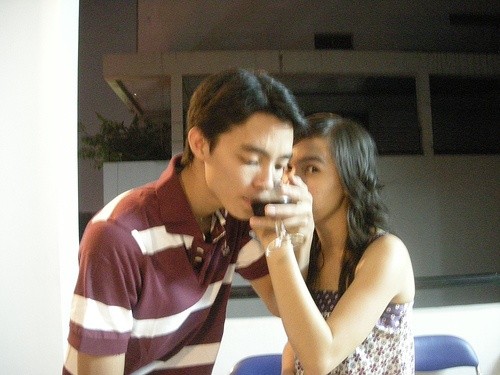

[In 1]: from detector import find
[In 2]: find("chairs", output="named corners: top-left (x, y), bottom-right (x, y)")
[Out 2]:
top-left (415, 335), bottom-right (479, 374)
top-left (229, 354), bottom-right (282, 374)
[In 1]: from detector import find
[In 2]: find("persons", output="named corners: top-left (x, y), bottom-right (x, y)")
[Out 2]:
top-left (54, 61), bottom-right (317, 375)
top-left (253, 110), bottom-right (417, 375)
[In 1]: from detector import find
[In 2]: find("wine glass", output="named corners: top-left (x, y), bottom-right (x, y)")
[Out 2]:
top-left (249, 172), bottom-right (308, 260)
